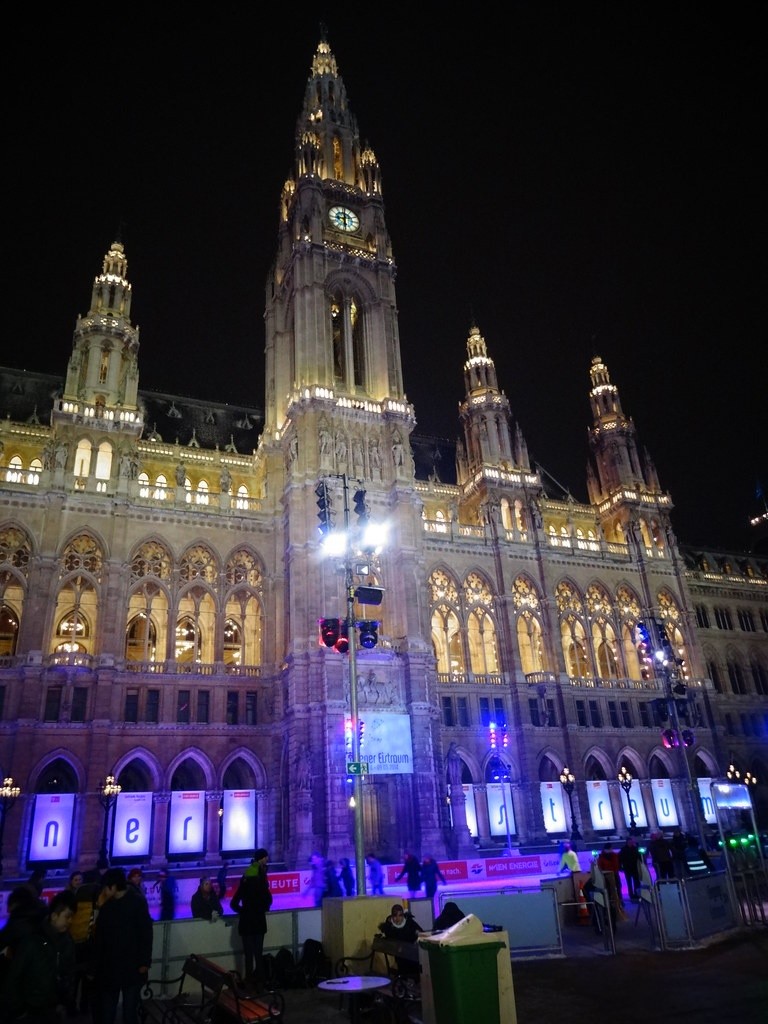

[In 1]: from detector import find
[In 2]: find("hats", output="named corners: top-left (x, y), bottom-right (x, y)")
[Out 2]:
top-left (128, 868), bottom-right (142, 879)
top-left (254, 848), bottom-right (269, 861)
top-left (244, 862), bottom-right (260, 877)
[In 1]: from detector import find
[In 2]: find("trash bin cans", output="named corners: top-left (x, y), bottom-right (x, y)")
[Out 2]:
top-left (417, 933), bottom-right (517, 1024)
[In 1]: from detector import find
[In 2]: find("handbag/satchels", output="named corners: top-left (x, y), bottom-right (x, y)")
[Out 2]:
top-left (371, 949), bottom-right (398, 976)
top-left (393, 967), bottom-right (421, 988)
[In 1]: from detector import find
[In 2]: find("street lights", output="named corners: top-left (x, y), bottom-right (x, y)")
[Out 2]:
top-left (617, 765), bottom-right (641, 836)
top-left (559, 765), bottom-right (584, 841)
top-left (320, 525), bottom-right (392, 896)
top-left (95, 770), bottom-right (123, 869)
top-left (654, 649), bottom-right (716, 838)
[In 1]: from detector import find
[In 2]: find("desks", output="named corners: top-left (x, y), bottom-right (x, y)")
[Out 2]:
top-left (318, 976), bottom-right (391, 1024)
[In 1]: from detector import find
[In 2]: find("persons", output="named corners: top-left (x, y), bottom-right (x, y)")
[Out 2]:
top-left (290, 425), bottom-right (406, 467)
top-left (366, 667), bottom-right (380, 695)
top-left (304, 850), bottom-right (448, 908)
top-left (378, 904), bottom-right (425, 983)
top-left (0, 866), bottom-right (153, 1024)
top-left (191, 861), bottom-right (228, 922)
top-left (219, 464), bottom-right (233, 493)
top-left (41, 440), bottom-right (68, 470)
top-left (158, 868), bottom-right (178, 920)
top-left (175, 460), bottom-right (186, 487)
top-left (556, 842), bottom-right (581, 877)
top-left (127, 450), bottom-right (139, 479)
top-left (231, 848), bottom-right (273, 981)
top-left (598, 826), bottom-right (690, 907)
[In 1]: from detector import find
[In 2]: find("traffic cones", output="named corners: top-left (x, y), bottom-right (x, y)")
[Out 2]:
top-left (578, 878), bottom-right (592, 927)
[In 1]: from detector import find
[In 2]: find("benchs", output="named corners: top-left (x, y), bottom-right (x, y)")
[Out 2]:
top-left (139, 954), bottom-right (286, 1024)
top-left (334, 934), bottom-right (422, 1024)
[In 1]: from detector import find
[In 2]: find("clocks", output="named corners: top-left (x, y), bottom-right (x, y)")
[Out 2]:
top-left (328, 204), bottom-right (360, 233)
